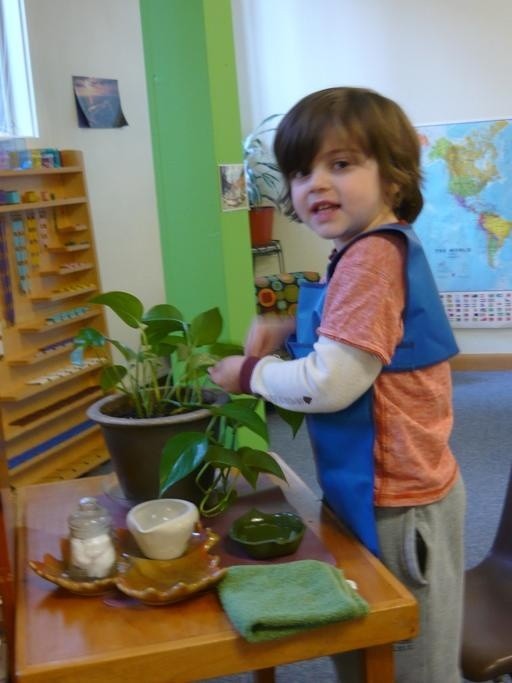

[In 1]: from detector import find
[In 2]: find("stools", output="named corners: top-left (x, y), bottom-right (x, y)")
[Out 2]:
top-left (251, 240), bottom-right (285, 275)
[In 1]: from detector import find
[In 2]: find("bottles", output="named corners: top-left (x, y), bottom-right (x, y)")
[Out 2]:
top-left (63, 493), bottom-right (117, 578)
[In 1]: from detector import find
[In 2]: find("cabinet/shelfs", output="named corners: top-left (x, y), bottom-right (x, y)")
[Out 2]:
top-left (0, 150), bottom-right (118, 484)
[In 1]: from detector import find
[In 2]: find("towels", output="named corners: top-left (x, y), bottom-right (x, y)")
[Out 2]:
top-left (214, 559), bottom-right (368, 644)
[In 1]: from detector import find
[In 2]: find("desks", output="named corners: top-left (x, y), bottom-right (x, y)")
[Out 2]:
top-left (13, 451), bottom-right (420, 683)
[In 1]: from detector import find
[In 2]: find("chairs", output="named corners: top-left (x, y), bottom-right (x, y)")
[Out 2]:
top-left (254, 272), bottom-right (320, 319)
top-left (458, 463), bottom-right (512, 683)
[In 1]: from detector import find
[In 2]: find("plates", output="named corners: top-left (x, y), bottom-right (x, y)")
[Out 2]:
top-left (27, 521), bottom-right (226, 603)
top-left (229, 507), bottom-right (305, 556)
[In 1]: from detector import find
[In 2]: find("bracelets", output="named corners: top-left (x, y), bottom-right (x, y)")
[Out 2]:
top-left (238, 355), bottom-right (260, 394)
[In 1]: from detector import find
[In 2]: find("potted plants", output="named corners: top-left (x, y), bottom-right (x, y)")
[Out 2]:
top-left (242, 113), bottom-right (288, 247)
top-left (70, 290), bottom-right (303, 516)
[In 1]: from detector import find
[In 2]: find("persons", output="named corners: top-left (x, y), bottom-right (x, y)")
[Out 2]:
top-left (202, 84), bottom-right (468, 682)
